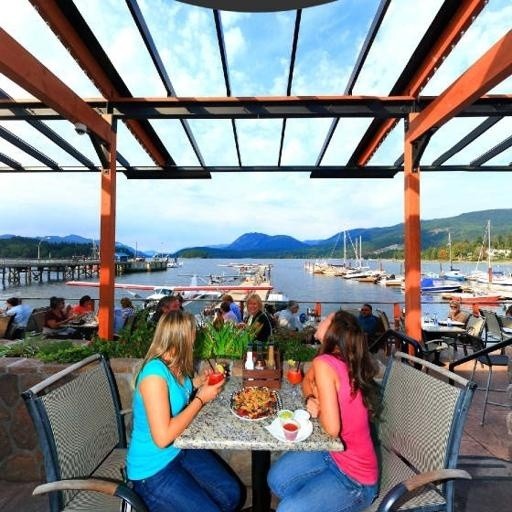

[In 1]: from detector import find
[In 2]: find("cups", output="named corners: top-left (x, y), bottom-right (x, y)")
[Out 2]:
top-left (206, 367), bottom-right (224, 384)
top-left (277, 407), bottom-right (311, 441)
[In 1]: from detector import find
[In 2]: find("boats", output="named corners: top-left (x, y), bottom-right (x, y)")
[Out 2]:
top-left (303, 223), bottom-right (512, 292)
top-left (217, 261), bottom-right (261, 273)
top-left (167, 261), bottom-right (184, 268)
top-left (266, 291), bottom-right (289, 311)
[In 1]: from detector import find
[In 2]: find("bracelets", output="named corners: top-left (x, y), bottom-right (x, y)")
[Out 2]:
top-left (195, 396), bottom-right (203, 406)
top-left (305, 394), bottom-right (316, 405)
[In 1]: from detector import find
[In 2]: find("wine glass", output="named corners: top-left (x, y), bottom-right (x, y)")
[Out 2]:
top-left (287, 366), bottom-right (305, 399)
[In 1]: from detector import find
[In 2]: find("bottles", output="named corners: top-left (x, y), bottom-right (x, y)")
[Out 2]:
top-left (244, 345), bottom-right (276, 380)
top-left (306, 307), bottom-right (317, 324)
top-left (423, 310), bottom-right (439, 327)
top-left (447, 316), bottom-right (453, 329)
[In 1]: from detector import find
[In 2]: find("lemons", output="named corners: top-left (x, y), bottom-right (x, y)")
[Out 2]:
top-left (214, 364), bottom-right (224, 374)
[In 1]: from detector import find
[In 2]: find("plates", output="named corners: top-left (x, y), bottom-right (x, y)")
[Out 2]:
top-left (271, 417), bottom-right (313, 441)
top-left (228, 394), bottom-right (268, 421)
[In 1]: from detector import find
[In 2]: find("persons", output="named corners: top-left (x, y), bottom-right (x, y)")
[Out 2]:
top-left (145, 293), bottom-right (306, 352)
top-left (127, 311), bottom-right (248, 512)
top-left (357, 304), bottom-right (380, 346)
top-left (1, 295), bottom-right (135, 340)
top-left (267, 309), bottom-right (386, 511)
top-left (447, 300), bottom-right (467, 328)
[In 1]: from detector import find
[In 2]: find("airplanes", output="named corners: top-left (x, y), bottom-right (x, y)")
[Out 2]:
top-left (65, 273), bottom-right (274, 319)
top-left (176, 271), bottom-right (260, 287)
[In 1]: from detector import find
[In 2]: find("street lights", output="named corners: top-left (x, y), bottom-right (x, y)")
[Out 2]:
top-left (37, 237), bottom-right (51, 259)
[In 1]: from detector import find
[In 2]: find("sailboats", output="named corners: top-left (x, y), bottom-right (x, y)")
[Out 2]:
top-left (440, 218), bottom-right (502, 305)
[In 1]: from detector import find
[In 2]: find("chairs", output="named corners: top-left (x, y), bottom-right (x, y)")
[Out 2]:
top-left (351, 347), bottom-right (477, 512)
top-left (373, 307), bottom-right (512, 428)
top-left (0, 308), bottom-right (148, 339)
top-left (19, 352), bottom-right (150, 512)
